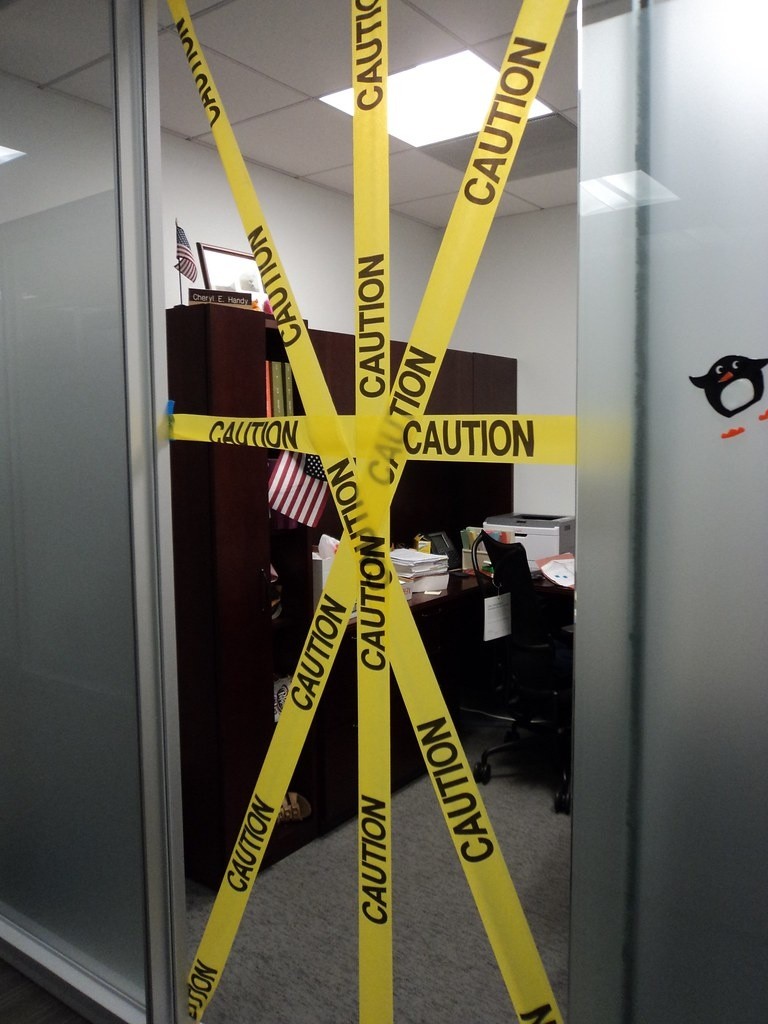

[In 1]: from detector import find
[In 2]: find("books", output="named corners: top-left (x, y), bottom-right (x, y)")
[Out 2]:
top-left (389, 547), bottom-right (449, 600)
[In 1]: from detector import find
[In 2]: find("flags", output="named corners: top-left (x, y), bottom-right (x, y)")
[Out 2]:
top-left (174, 224), bottom-right (198, 284)
top-left (266, 444), bottom-right (330, 529)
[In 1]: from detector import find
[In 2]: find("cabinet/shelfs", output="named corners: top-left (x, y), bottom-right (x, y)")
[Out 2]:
top-left (309, 330), bottom-right (517, 545)
top-left (165, 303), bottom-right (309, 894)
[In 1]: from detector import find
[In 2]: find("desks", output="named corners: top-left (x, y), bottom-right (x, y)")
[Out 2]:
top-left (317, 570), bottom-right (575, 836)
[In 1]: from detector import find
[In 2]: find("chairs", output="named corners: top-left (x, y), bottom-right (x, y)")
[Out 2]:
top-left (472, 531), bottom-right (572, 816)
